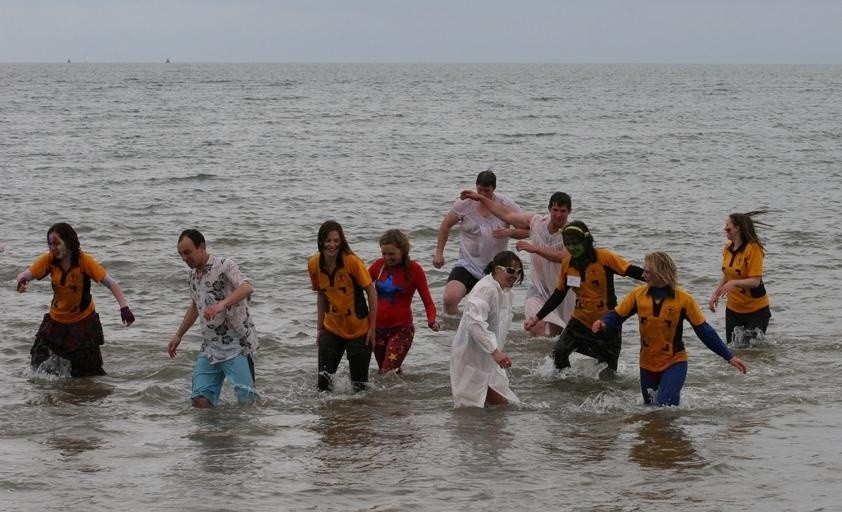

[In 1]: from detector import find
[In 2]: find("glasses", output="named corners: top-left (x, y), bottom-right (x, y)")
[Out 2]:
top-left (498, 265), bottom-right (522, 274)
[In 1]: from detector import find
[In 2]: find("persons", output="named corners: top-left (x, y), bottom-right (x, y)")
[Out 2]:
top-left (449, 249), bottom-right (525, 409)
top-left (432, 171), bottom-right (530, 317)
top-left (166, 228), bottom-right (260, 409)
top-left (460, 189), bottom-right (576, 338)
top-left (306, 217), bottom-right (379, 395)
top-left (524, 219), bottom-right (646, 379)
top-left (14, 220), bottom-right (136, 375)
top-left (708, 211), bottom-right (772, 347)
top-left (591, 251), bottom-right (749, 408)
top-left (366, 226), bottom-right (440, 382)
